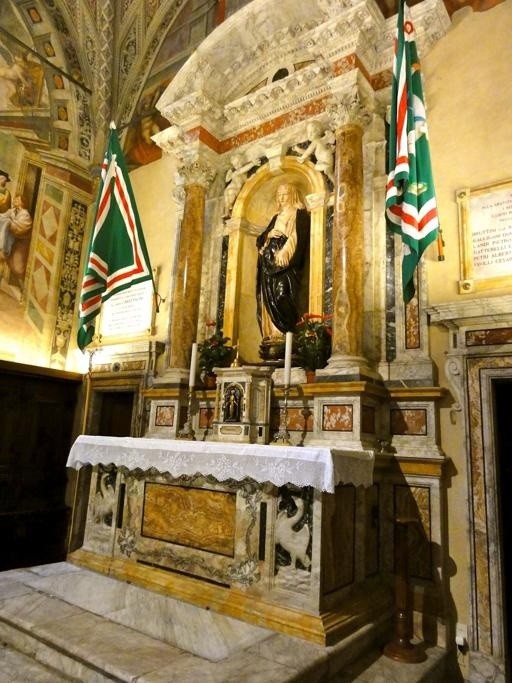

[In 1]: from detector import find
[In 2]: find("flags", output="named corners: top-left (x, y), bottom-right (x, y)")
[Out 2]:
top-left (385, 0), bottom-right (440, 304)
top-left (75, 121), bottom-right (152, 353)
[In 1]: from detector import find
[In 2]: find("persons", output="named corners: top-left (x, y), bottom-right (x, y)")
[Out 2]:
top-left (220, 152), bottom-right (262, 219)
top-left (0, 193), bottom-right (32, 288)
top-left (0, 168), bottom-right (12, 213)
top-left (292, 120), bottom-right (336, 187)
top-left (255, 183), bottom-right (309, 338)
top-left (228, 389), bottom-right (238, 419)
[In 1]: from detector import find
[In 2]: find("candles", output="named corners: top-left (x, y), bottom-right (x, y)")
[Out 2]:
top-left (285, 330), bottom-right (293, 382)
top-left (188, 341), bottom-right (197, 387)
